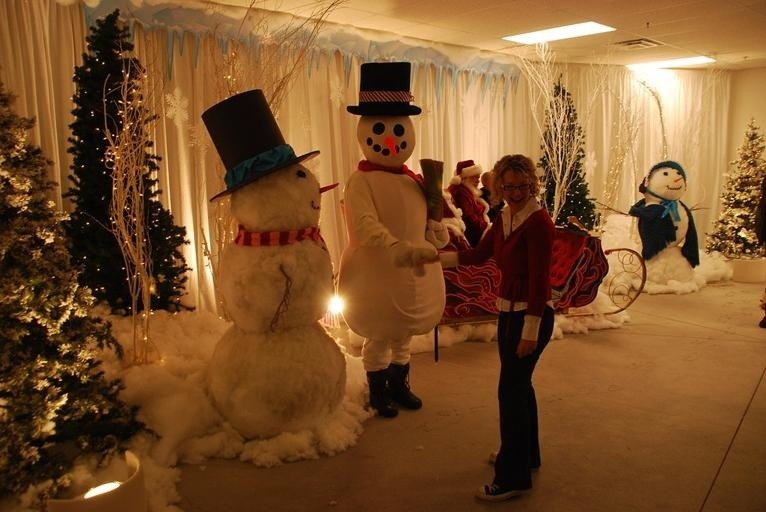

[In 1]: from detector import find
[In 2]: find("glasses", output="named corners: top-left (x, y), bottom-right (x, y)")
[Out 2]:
top-left (501, 182), bottom-right (531, 191)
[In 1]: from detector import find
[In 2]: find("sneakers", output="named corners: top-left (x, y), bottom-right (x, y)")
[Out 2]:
top-left (490, 450), bottom-right (539, 473)
top-left (476, 483), bottom-right (531, 502)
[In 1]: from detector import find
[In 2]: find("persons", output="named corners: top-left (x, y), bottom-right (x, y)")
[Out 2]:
top-left (447, 154), bottom-right (555, 500)
top-left (443, 157), bottom-right (495, 246)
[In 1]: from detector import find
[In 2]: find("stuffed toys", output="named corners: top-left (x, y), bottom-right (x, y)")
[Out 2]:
top-left (336, 61), bottom-right (466, 415)
top-left (626, 160), bottom-right (714, 269)
top-left (203, 88), bottom-right (341, 440)
